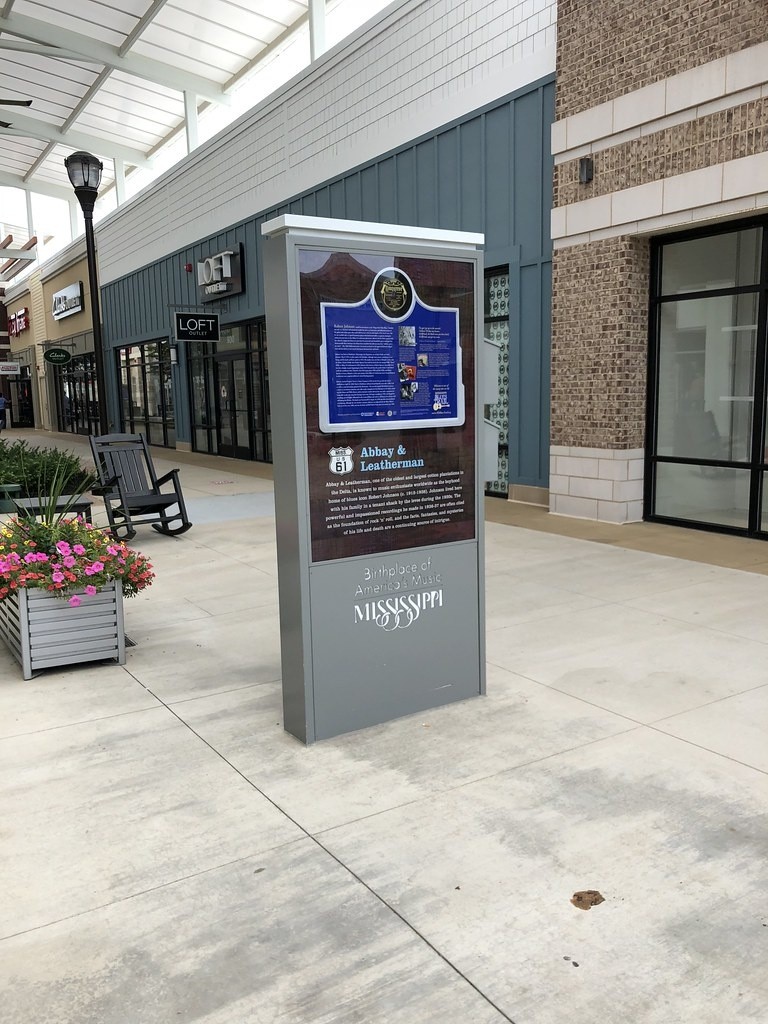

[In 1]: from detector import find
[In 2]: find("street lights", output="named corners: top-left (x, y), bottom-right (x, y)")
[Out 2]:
top-left (65, 152), bottom-right (120, 494)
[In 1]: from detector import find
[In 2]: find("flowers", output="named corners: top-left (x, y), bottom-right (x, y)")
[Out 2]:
top-left (0, 444), bottom-right (156, 598)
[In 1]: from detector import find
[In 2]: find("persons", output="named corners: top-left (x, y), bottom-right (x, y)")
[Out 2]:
top-left (402, 387), bottom-right (411, 400)
top-left (0, 393), bottom-right (11, 429)
top-left (411, 383), bottom-right (418, 392)
top-left (418, 359), bottom-right (425, 367)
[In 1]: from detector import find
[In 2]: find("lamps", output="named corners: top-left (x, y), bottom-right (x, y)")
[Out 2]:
top-left (169, 343), bottom-right (179, 365)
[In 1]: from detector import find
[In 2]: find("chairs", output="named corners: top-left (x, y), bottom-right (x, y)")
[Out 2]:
top-left (89, 431), bottom-right (192, 544)
top-left (686, 412), bottom-right (748, 481)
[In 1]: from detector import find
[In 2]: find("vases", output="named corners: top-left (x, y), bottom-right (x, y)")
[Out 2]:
top-left (0, 577), bottom-right (126, 681)
